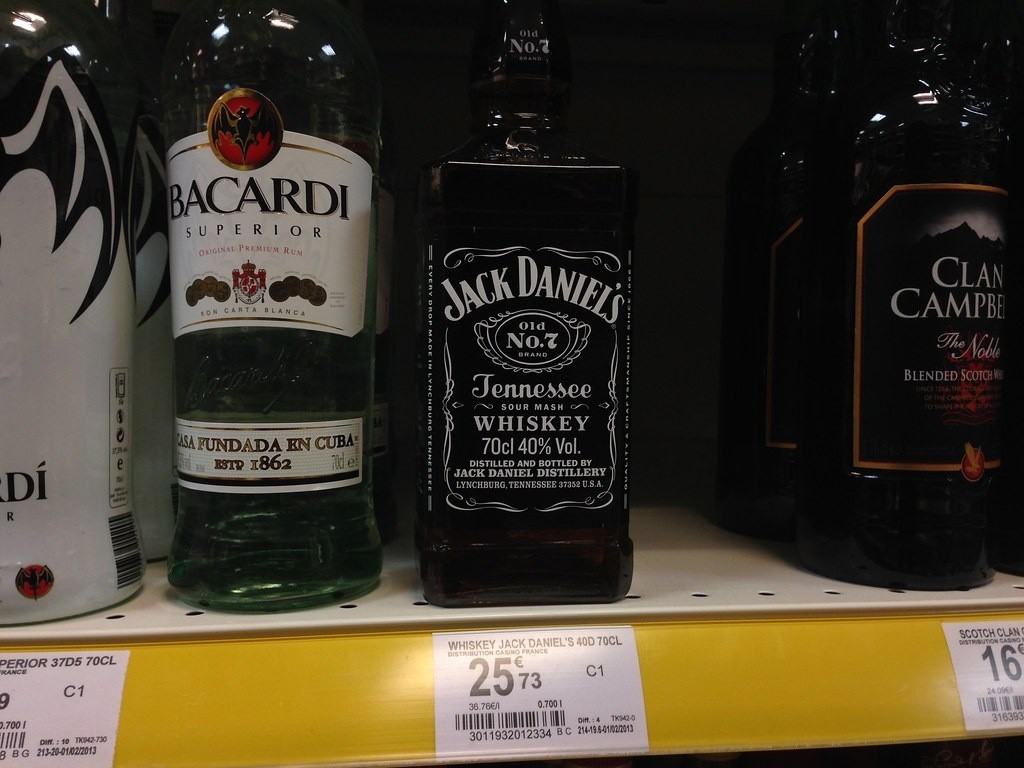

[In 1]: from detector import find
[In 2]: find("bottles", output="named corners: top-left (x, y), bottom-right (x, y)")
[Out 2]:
top-left (417, 0), bottom-right (635, 608)
top-left (717, 0), bottom-right (1024, 590)
top-left (168, 0), bottom-right (399, 609)
top-left (0, 0), bottom-right (168, 624)
